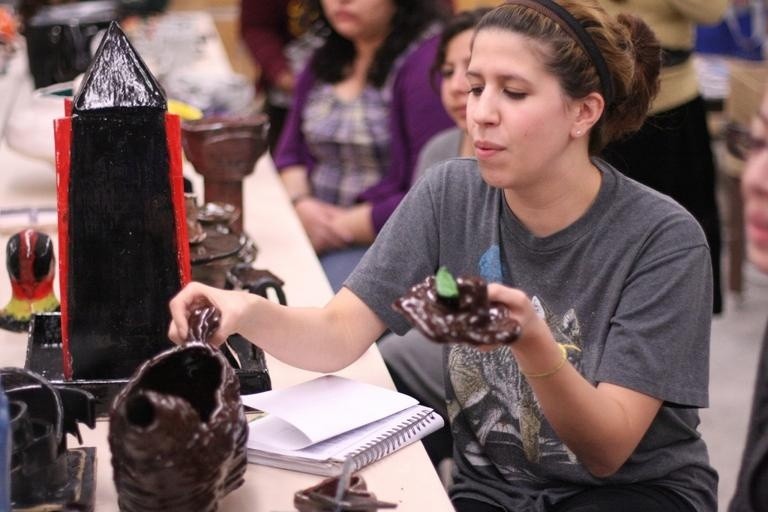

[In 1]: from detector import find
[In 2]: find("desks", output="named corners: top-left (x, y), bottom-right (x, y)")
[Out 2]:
top-left (2, 12), bottom-right (451, 512)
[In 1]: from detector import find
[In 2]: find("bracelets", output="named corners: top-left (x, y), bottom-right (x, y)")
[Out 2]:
top-left (515, 343), bottom-right (582, 381)
top-left (292, 190), bottom-right (314, 207)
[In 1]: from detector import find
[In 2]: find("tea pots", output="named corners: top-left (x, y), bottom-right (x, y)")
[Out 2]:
top-left (107, 302), bottom-right (248, 511)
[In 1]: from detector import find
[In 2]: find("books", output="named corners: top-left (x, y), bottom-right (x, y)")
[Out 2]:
top-left (238, 373), bottom-right (449, 479)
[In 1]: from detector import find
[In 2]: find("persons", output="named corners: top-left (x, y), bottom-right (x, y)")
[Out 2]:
top-left (575, 1), bottom-right (766, 316)
top-left (721, 77), bottom-right (767, 512)
top-left (273, 2), bottom-right (455, 289)
top-left (374, 6), bottom-right (497, 458)
top-left (241, 1), bottom-right (332, 158)
top-left (166, 0), bottom-right (720, 511)
